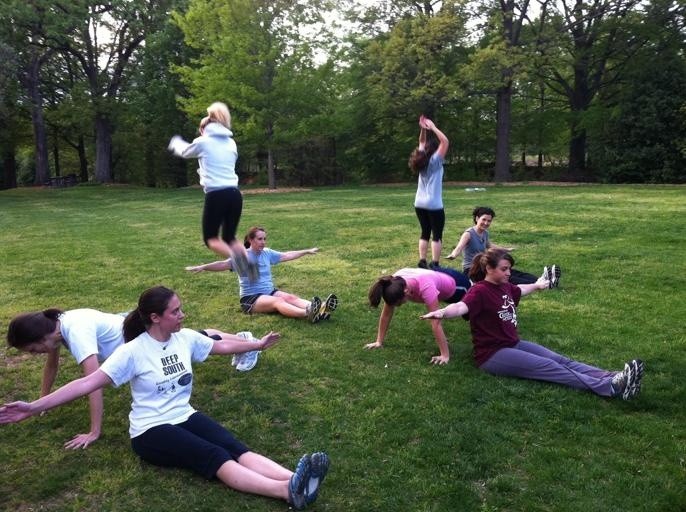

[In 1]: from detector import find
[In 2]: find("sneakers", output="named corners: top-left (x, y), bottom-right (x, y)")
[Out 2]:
top-left (305, 452), bottom-right (329, 503)
top-left (418, 259), bottom-right (428, 269)
top-left (231, 332), bottom-right (253, 367)
top-left (306, 296), bottom-right (321, 323)
top-left (541, 266), bottom-right (552, 290)
top-left (236, 337), bottom-right (258, 371)
top-left (290, 454), bottom-right (310, 509)
top-left (551, 264), bottom-right (560, 287)
top-left (319, 294), bottom-right (337, 320)
top-left (429, 261), bottom-right (439, 270)
top-left (633, 358), bottom-right (644, 397)
top-left (613, 362), bottom-right (635, 400)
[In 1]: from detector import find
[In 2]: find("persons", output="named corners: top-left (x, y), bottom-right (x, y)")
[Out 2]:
top-left (445, 206), bottom-right (562, 290)
top-left (359, 267), bottom-right (484, 365)
top-left (168, 101), bottom-right (259, 282)
top-left (6, 310), bottom-right (262, 451)
top-left (418, 247), bottom-right (646, 400)
top-left (0, 285), bottom-right (331, 510)
top-left (406, 115), bottom-right (451, 269)
top-left (186, 227), bottom-right (340, 322)
